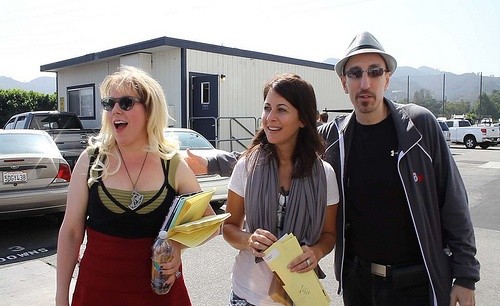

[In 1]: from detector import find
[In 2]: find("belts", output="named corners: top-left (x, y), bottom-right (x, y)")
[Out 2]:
top-left (347, 257), bottom-right (401, 280)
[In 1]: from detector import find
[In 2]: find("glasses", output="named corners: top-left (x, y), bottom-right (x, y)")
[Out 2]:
top-left (101, 96), bottom-right (146, 111)
top-left (344, 67), bottom-right (388, 78)
top-left (275, 186), bottom-right (286, 230)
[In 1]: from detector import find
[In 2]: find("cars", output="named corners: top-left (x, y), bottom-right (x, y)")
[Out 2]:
top-left (0, 127), bottom-right (71, 231)
top-left (437, 120), bottom-right (452, 148)
top-left (160, 127), bottom-right (232, 211)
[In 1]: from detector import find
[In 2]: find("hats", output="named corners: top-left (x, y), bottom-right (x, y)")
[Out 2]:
top-left (335, 31), bottom-right (397, 80)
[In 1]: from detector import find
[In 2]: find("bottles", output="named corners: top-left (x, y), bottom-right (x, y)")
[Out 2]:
top-left (151, 230), bottom-right (173, 294)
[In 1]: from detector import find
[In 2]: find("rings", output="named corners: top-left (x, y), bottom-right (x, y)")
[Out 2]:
top-left (306, 259), bottom-right (310, 267)
top-left (174, 272), bottom-right (182, 278)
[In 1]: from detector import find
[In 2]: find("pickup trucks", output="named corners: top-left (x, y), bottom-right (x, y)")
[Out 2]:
top-left (443, 119), bottom-right (500, 150)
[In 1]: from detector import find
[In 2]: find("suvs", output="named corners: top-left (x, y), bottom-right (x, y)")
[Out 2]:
top-left (2, 109), bottom-right (90, 169)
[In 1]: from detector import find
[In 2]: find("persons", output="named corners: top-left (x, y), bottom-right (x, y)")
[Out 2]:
top-left (113, 138), bottom-right (149, 210)
top-left (56, 65), bottom-right (221, 306)
top-left (186, 31), bottom-right (480, 306)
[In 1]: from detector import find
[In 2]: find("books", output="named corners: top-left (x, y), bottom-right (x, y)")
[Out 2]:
top-left (155, 188), bottom-right (231, 248)
